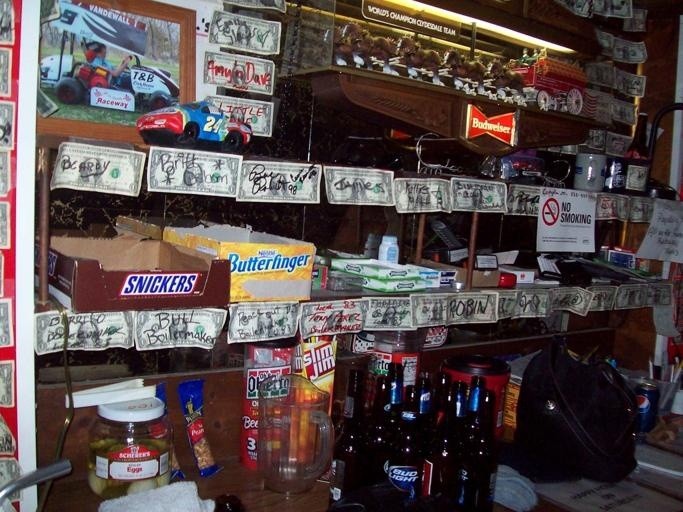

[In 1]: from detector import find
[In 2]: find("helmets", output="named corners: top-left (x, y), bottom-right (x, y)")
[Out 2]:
top-left (513, 334), bottom-right (641, 485)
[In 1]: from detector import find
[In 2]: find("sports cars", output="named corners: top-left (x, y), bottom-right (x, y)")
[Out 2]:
top-left (27, 49), bottom-right (182, 112)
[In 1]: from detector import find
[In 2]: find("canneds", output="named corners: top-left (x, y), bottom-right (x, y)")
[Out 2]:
top-left (86, 396), bottom-right (172, 499)
top-left (635, 382), bottom-right (659, 433)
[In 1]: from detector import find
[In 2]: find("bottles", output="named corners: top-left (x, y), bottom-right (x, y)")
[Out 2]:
top-left (625, 112), bottom-right (654, 195)
top-left (328, 330), bottom-right (501, 512)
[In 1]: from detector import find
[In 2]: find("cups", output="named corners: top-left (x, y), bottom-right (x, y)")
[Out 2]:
top-left (572, 153), bottom-right (608, 193)
top-left (257, 373), bottom-right (336, 497)
top-left (652, 378), bottom-right (683, 414)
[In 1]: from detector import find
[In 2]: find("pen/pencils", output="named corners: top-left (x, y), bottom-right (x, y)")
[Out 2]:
top-left (647, 355), bottom-right (683, 411)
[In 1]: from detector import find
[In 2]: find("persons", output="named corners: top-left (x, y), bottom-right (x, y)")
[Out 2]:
top-left (89, 42), bottom-right (136, 90)
